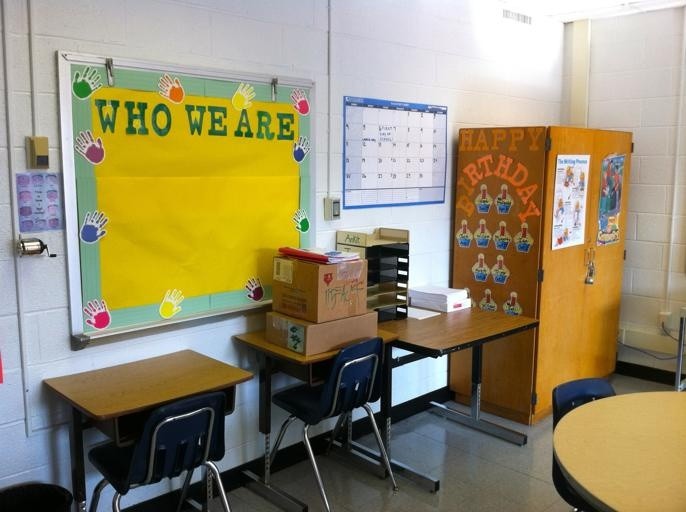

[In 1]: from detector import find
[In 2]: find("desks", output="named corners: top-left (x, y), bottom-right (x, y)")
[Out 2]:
top-left (553, 392), bottom-right (686, 512)
top-left (233, 328), bottom-right (400, 511)
top-left (351, 306), bottom-right (539, 492)
top-left (44, 350), bottom-right (254, 512)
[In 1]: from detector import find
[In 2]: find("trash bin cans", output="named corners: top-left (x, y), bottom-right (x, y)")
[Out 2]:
top-left (0, 481), bottom-right (74, 512)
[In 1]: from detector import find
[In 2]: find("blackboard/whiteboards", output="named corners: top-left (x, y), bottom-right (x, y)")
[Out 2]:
top-left (54, 50), bottom-right (317, 352)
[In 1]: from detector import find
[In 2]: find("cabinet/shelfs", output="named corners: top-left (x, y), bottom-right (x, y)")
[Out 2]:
top-left (335, 226), bottom-right (409, 322)
top-left (448, 125), bottom-right (635, 427)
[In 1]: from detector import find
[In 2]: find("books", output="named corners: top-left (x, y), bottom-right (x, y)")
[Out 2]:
top-left (279, 247), bottom-right (359, 262)
top-left (410, 288), bottom-right (467, 302)
top-left (411, 300), bottom-right (471, 313)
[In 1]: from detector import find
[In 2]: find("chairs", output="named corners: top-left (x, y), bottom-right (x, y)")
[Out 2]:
top-left (260, 335), bottom-right (397, 511)
top-left (88, 390), bottom-right (229, 512)
top-left (552, 378), bottom-right (616, 431)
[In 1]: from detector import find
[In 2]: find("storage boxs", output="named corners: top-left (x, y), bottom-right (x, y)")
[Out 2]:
top-left (265, 256), bottom-right (379, 356)
